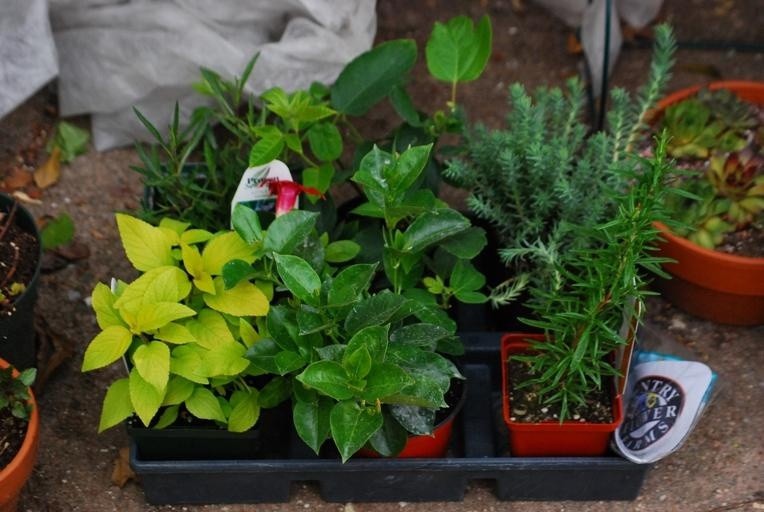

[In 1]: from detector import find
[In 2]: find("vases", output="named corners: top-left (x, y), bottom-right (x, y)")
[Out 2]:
top-left (1, 192), bottom-right (43, 368)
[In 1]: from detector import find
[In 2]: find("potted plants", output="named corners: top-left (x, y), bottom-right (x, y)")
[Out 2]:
top-left (313, 40), bottom-right (447, 230)
top-left (622, 81), bottom-right (764, 327)
top-left (222, 140), bottom-right (489, 463)
top-left (440, 20), bottom-right (677, 333)
top-left (500, 130), bottom-right (704, 457)
top-left (77, 213), bottom-right (271, 463)
top-left (1, 359), bottom-right (41, 510)
top-left (247, 14), bottom-right (495, 327)
top-left (137, 47), bottom-right (285, 281)
